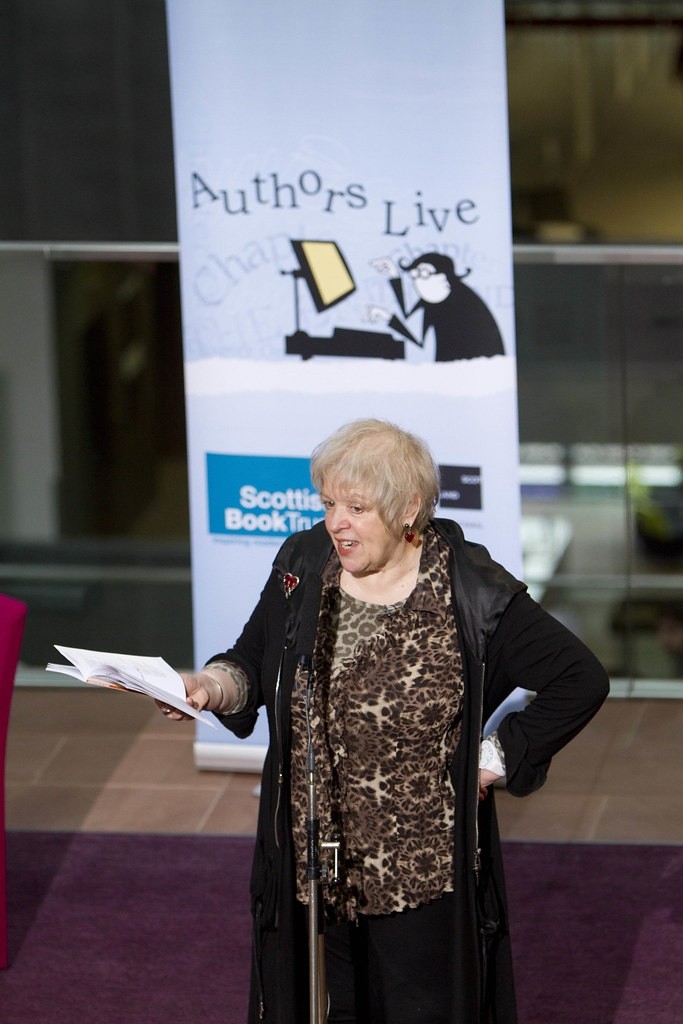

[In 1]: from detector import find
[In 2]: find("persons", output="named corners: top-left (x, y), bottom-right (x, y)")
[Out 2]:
top-left (154, 420), bottom-right (609, 1024)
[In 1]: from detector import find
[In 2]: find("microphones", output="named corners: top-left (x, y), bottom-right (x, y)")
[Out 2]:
top-left (296, 571), bottom-right (322, 665)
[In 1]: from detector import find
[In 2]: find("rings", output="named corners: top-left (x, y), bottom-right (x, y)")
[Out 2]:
top-left (163, 709), bottom-right (171, 715)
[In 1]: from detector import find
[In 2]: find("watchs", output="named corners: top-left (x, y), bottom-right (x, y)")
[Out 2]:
top-left (479, 740), bottom-right (507, 777)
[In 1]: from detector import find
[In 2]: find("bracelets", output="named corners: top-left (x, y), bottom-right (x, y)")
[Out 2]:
top-left (207, 675), bottom-right (223, 711)
